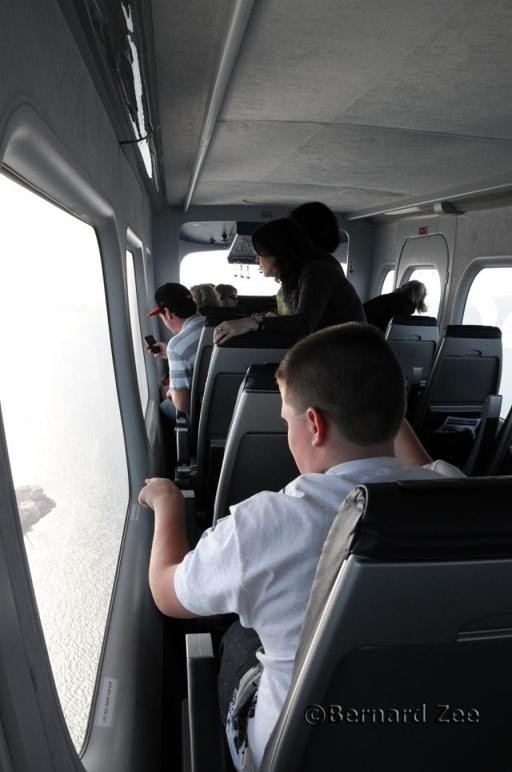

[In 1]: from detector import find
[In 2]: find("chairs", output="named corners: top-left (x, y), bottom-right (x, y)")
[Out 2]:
top-left (176, 305), bottom-right (512, 770)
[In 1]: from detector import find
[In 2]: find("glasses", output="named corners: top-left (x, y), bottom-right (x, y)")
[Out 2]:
top-left (225, 294), bottom-right (238, 301)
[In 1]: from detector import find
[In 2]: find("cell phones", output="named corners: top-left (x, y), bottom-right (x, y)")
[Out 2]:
top-left (144, 335), bottom-right (161, 354)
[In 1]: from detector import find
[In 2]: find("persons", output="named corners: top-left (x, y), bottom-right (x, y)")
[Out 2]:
top-left (205, 215), bottom-right (367, 349)
top-left (136, 319), bottom-right (472, 771)
top-left (188, 283), bottom-right (223, 311)
top-left (214, 284), bottom-right (240, 309)
top-left (351, 280), bottom-right (428, 335)
top-left (142, 281), bottom-right (211, 428)
top-left (282, 199), bottom-right (346, 259)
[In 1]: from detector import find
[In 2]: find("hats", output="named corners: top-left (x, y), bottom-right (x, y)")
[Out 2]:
top-left (145, 283), bottom-right (192, 317)
